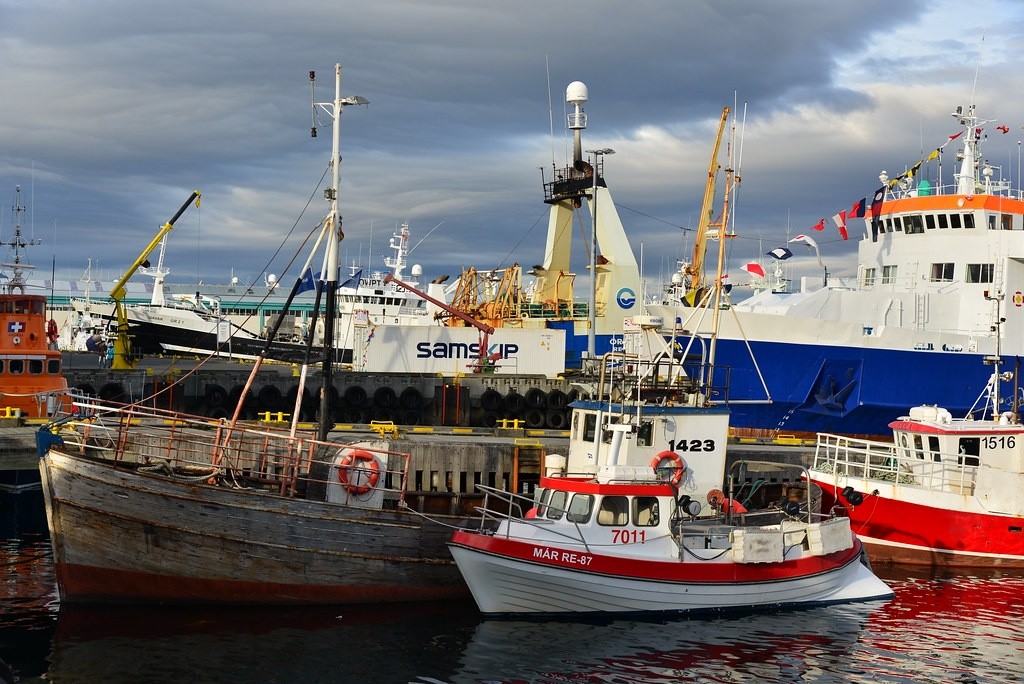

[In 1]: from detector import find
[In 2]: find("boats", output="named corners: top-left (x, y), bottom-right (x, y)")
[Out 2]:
top-left (0, 56), bottom-right (1024, 621)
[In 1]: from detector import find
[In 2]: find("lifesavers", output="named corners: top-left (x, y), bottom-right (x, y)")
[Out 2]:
top-left (338, 450), bottom-right (380, 495)
top-left (651, 451), bottom-right (685, 485)
top-left (48, 319), bottom-right (58, 342)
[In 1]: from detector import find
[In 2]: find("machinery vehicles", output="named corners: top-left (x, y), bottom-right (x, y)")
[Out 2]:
top-left (104, 188), bottom-right (204, 371)
top-left (684, 106), bottom-right (731, 307)
top-left (383, 271), bottom-right (503, 374)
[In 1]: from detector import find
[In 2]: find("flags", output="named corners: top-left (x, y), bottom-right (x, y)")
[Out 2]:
top-left (765, 247), bottom-right (794, 260)
top-left (740, 259), bottom-right (768, 285)
top-left (848, 197), bottom-right (866, 218)
top-left (788, 234), bottom-right (824, 268)
top-left (294, 267), bottom-right (362, 296)
top-left (871, 185), bottom-right (887, 242)
top-left (715, 274), bottom-right (733, 297)
top-left (994, 124), bottom-right (1011, 135)
top-left (679, 286), bottom-right (708, 308)
top-left (831, 209), bottom-right (849, 241)
top-left (810, 217), bottom-right (831, 231)
